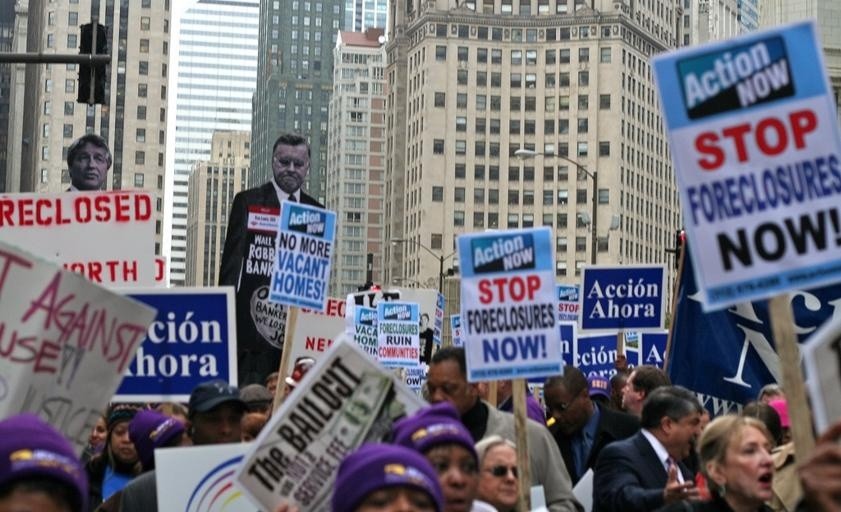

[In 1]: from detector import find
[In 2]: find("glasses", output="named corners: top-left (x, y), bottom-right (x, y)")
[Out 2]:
top-left (493, 465), bottom-right (517, 479)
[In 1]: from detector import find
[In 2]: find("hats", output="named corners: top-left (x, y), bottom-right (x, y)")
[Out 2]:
top-left (586, 375), bottom-right (613, 401)
top-left (332, 442), bottom-right (445, 512)
top-left (105, 399), bottom-right (152, 434)
top-left (188, 380), bottom-right (245, 415)
top-left (240, 383), bottom-right (275, 405)
top-left (127, 410), bottom-right (185, 460)
top-left (0, 412), bottom-right (89, 512)
top-left (390, 399), bottom-right (480, 462)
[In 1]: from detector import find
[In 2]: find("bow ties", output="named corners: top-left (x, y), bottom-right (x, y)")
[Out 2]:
top-left (666, 457), bottom-right (682, 486)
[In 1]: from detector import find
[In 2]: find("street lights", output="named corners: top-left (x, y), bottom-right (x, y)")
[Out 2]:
top-left (391, 237), bottom-right (445, 295)
top-left (514, 148), bottom-right (599, 266)
top-left (392, 275), bottom-right (427, 289)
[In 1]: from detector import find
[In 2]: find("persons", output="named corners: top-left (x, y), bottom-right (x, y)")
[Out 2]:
top-left (2, 348), bottom-right (840, 510)
top-left (419, 312), bottom-right (434, 362)
top-left (219, 135), bottom-right (326, 389)
top-left (64, 134), bottom-right (112, 191)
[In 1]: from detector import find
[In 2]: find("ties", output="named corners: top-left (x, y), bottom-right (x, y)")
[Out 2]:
top-left (579, 431), bottom-right (591, 472)
